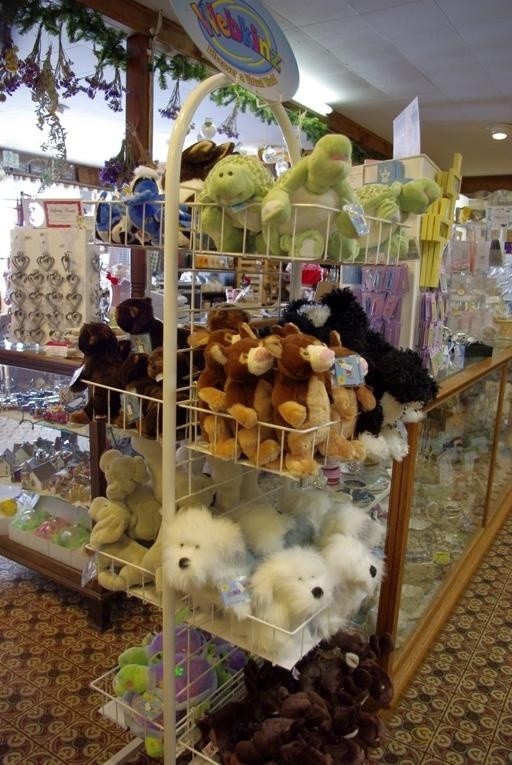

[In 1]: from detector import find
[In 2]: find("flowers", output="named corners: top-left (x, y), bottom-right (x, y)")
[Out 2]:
top-left (216, 97), bottom-right (244, 140)
top-left (95, 134), bottom-right (136, 184)
top-left (0, 2), bottom-right (130, 191)
top-left (157, 62), bottom-right (188, 121)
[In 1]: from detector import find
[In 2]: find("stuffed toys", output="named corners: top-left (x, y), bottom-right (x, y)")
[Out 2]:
top-left (281, 287), bottom-right (441, 462)
top-left (83, 439), bottom-right (259, 595)
top-left (258, 134), bottom-right (364, 262)
top-left (264, 321), bottom-right (357, 478)
top-left (90, 138), bottom-right (235, 248)
top-left (326, 330), bottom-right (377, 464)
top-left (164, 487), bottom-right (385, 649)
top-left (205, 323), bottom-right (281, 466)
top-left (356, 179), bottom-right (442, 261)
top-left (187, 325), bottom-right (242, 461)
top-left (67, 295), bottom-right (191, 437)
top-left (201, 631), bottom-right (395, 764)
top-left (195, 152), bottom-right (276, 259)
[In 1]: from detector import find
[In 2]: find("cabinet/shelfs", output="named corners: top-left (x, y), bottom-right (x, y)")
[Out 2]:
top-left (0, 296), bottom-right (115, 633)
top-left (90, 213), bottom-right (512, 765)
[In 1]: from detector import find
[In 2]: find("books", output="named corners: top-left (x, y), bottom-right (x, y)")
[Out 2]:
top-left (111, 626), bottom-right (241, 755)
